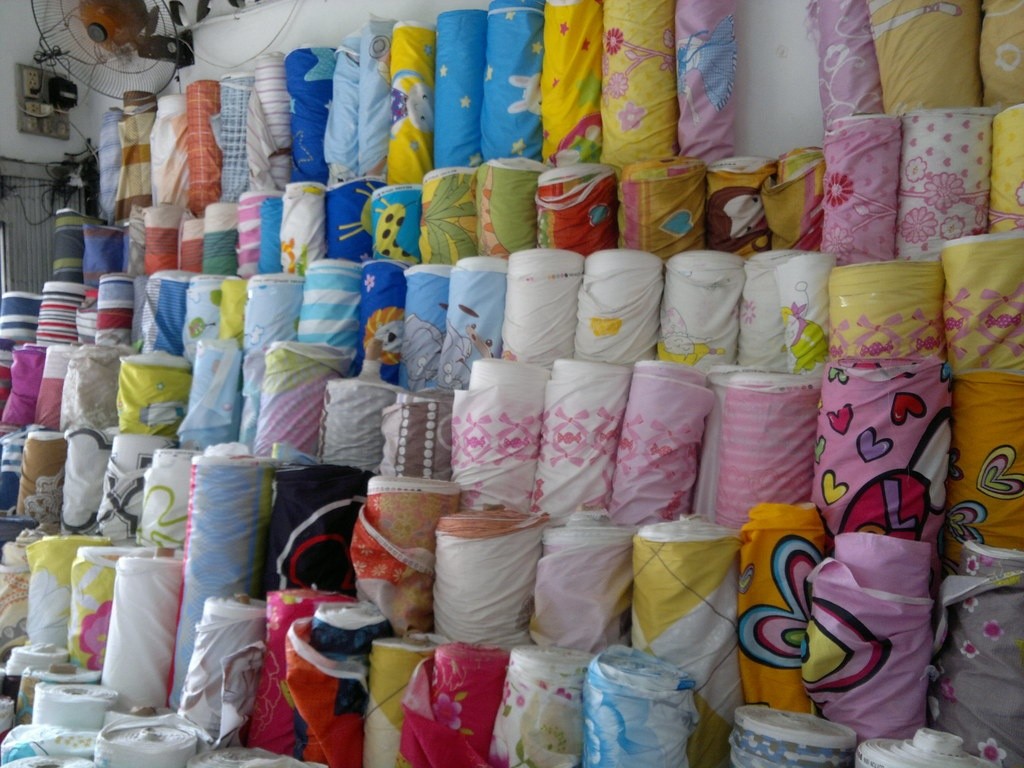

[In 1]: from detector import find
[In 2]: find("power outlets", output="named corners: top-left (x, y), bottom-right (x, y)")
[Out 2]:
top-left (25, 101), bottom-right (54, 118)
top-left (23, 68), bottom-right (40, 100)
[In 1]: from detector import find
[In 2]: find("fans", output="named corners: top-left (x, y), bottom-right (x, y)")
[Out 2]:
top-left (30, 0), bottom-right (195, 101)
